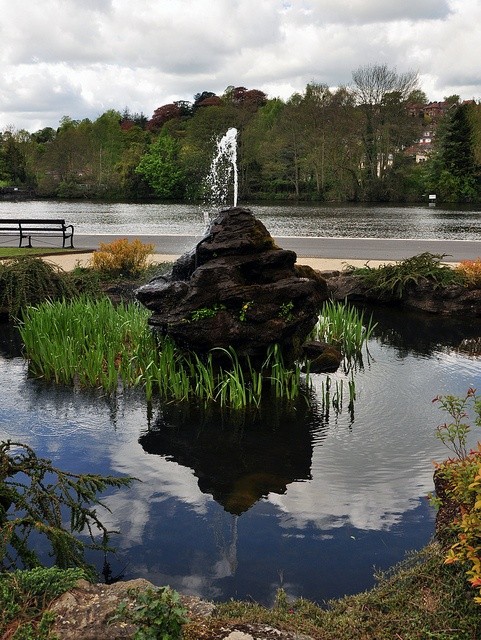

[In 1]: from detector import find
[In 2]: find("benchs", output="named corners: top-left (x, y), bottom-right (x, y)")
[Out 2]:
top-left (0, 218), bottom-right (74, 249)
